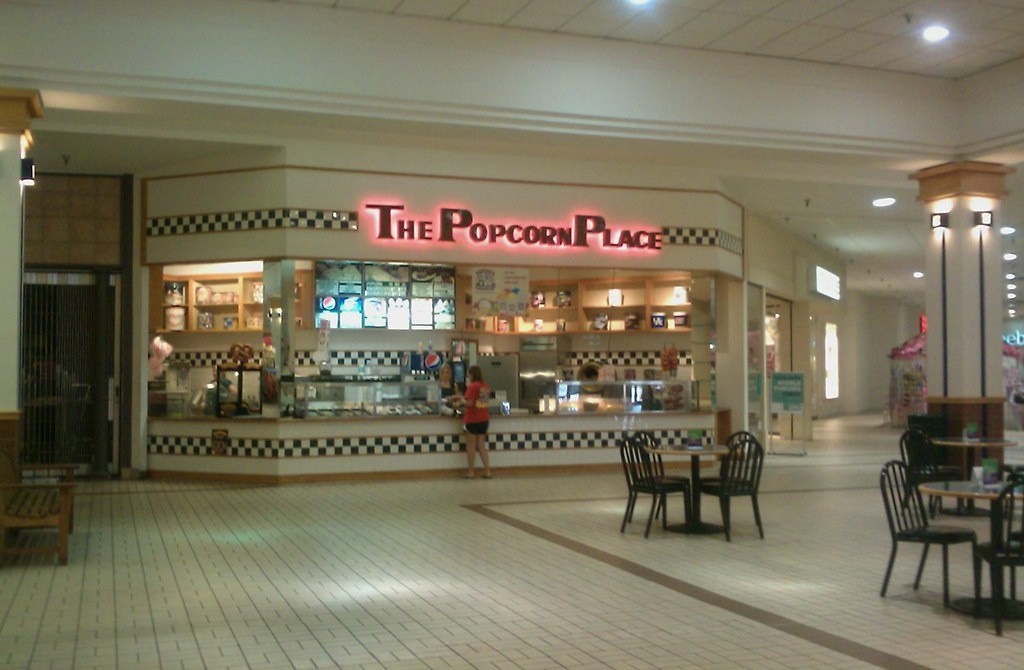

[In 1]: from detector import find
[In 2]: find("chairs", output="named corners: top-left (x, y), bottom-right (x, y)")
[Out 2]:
top-left (880, 459), bottom-right (980, 609)
top-left (618, 430), bottom-right (765, 542)
top-left (899, 429), bottom-right (966, 521)
top-left (975, 481), bottom-right (1024, 636)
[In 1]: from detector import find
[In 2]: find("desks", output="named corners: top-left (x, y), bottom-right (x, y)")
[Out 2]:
top-left (930, 436), bottom-right (1019, 519)
top-left (646, 446), bottom-right (731, 535)
top-left (920, 482), bottom-right (1024, 623)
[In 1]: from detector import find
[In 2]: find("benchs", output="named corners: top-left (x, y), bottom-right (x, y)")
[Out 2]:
top-left (0, 449), bottom-right (81, 567)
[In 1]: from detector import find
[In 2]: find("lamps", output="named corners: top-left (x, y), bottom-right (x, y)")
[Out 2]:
top-left (931, 212), bottom-right (948, 228)
top-left (974, 211), bottom-right (993, 229)
top-left (22, 157), bottom-right (36, 186)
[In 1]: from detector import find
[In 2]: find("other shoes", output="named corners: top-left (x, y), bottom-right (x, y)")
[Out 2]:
top-left (462, 475), bottom-right (476, 479)
top-left (482, 475), bottom-right (492, 479)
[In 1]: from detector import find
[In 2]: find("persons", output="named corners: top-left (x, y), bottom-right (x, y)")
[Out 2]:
top-left (426, 363), bottom-right (461, 411)
top-left (461, 365), bottom-right (494, 479)
top-left (205, 365), bottom-right (237, 403)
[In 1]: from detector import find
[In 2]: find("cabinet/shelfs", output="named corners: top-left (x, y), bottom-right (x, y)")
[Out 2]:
top-left (580, 274), bottom-right (691, 334)
top-left (455, 274), bottom-right (494, 334)
top-left (495, 279), bottom-right (582, 336)
top-left (161, 269), bottom-right (311, 332)
